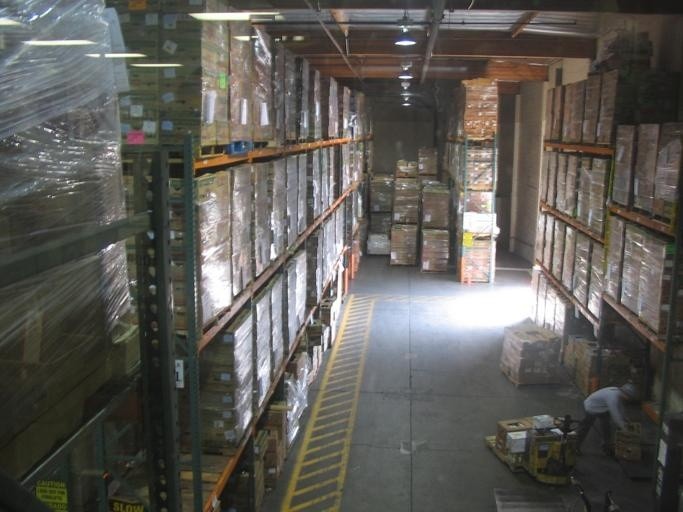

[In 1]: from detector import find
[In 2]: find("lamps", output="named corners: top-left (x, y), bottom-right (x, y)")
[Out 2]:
top-left (394, 30), bottom-right (416, 47)
top-left (402, 83), bottom-right (409, 90)
top-left (398, 69), bottom-right (413, 80)
top-left (400, 100), bottom-right (414, 107)
top-left (401, 91), bottom-right (414, 97)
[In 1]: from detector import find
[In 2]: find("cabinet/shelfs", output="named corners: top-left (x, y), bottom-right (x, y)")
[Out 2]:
top-left (435, 80), bottom-right (499, 285)
top-left (6, 5), bottom-right (375, 512)
top-left (531, 72), bottom-right (683, 511)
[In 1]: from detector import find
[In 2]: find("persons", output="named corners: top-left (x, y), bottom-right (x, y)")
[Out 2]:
top-left (576, 381), bottom-right (637, 454)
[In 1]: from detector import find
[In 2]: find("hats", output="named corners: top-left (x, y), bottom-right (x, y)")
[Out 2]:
top-left (621, 383), bottom-right (635, 398)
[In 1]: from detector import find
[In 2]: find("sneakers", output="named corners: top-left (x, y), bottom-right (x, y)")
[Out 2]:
top-left (576, 449), bottom-right (581, 456)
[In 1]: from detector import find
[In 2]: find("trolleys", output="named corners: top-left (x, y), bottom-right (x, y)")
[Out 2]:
top-left (483, 413), bottom-right (583, 488)
